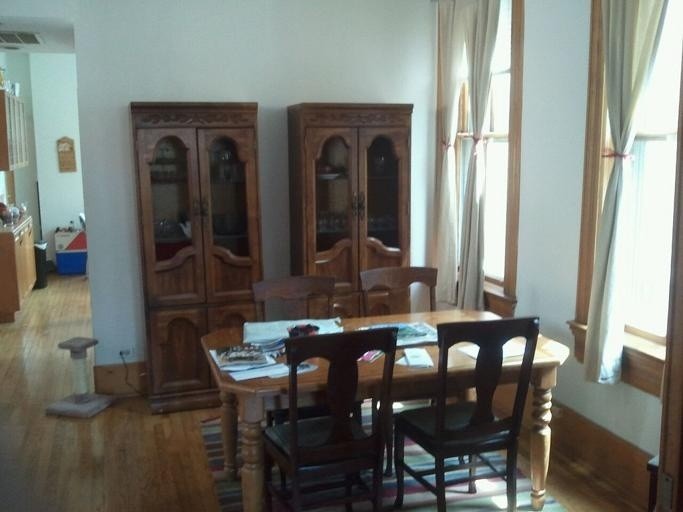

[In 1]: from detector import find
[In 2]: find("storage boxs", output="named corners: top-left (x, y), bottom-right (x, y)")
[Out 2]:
top-left (55, 249), bottom-right (89, 275)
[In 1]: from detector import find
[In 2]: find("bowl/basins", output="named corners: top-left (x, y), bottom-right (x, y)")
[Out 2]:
top-left (290, 324), bottom-right (319, 336)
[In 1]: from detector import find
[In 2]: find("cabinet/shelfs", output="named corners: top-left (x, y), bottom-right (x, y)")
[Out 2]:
top-left (127, 101), bottom-right (266, 416)
top-left (285, 102), bottom-right (415, 319)
top-left (1, 213), bottom-right (38, 325)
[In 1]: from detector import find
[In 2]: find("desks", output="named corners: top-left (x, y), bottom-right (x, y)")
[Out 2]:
top-left (199, 308), bottom-right (572, 511)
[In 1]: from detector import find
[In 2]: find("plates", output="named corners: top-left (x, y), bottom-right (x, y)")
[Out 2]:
top-left (319, 172), bottom-right (338, 179)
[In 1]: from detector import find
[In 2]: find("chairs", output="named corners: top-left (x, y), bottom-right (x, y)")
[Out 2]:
top-left (393, 316), bottom-right (544, 512)
top-left (251, 274), bottom-right (338, 323)
top-left (358, 266), bottom-right (477, 495)
top-left (261, 326), bottom-right (400, 511)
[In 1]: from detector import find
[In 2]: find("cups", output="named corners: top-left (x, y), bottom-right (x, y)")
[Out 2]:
top-left (3, 210), bottom-right (14, 226)
